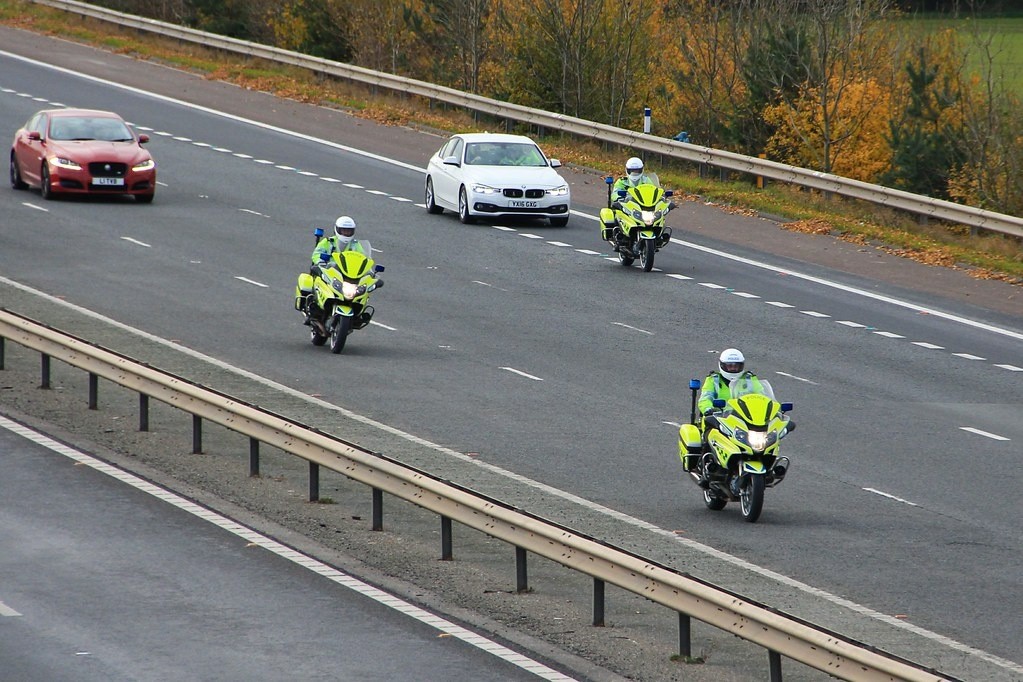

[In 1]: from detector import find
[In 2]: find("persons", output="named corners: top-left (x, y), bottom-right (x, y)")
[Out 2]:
top-left (611, 157), bottom-right (654, 253)
top-left (697, 347), bottom-right (765, 474)
top-left (469, 144), bottom-right (530, 166)
top-left (301, 215), bottom-right (367, 325)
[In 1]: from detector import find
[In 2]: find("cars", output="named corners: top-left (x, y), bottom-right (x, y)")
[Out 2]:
top-left (10, 108), bottom-right (156, 201)
top-left (424, 131), bottom-right (571, 226)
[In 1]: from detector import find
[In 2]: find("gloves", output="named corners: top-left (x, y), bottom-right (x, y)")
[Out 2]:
top-left (613, 203), bottom-right (622, 209)
top-left (669, 203), bottom-right (675, 209)
top-left (318, 261), bottom-right (327, 267)
top-left (704, 408), bottom-right (716, 415)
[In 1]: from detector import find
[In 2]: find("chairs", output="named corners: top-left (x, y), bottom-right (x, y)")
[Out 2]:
top-left (54, 121), bottom-right (72, 137)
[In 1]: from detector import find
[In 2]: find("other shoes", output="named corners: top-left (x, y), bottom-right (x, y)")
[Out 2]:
top-left (303, 316), bottom-right (310, 325)
top-left (697, 476), bottom-right (709, 488)
top-left (612, 246), bottom-right (617, 250)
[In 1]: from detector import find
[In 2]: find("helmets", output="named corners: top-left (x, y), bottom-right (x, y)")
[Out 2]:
top-left (334, 216), bottom-right (355, 243)
top-left (719, 348), bottom-right (746, 381)
top-left (624, 157), bottom-right (644, 181)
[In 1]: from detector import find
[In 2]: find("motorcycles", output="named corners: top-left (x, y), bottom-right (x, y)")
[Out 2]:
top-left (599, 172), bottom-right (675, 272)
top-left (678, 378), bottom-right (796, 522)
top-left (295, 228), bottom-right (385, 353)
